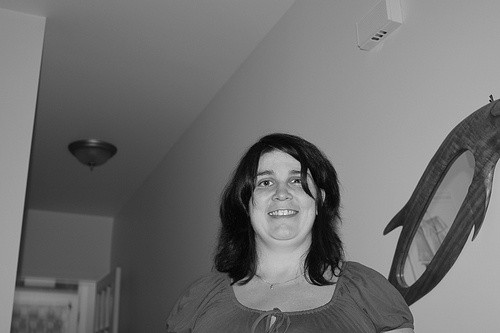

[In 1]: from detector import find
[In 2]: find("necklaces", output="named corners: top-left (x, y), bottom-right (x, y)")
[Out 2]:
top-left (255, 271), bottom-right (308, 290)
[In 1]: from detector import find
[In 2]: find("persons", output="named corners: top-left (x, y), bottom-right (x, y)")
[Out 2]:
top-left (164, 133), bottom-right (414, 333)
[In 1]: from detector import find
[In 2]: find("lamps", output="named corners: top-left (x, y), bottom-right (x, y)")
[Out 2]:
top-left (68, 140), bottom-right (117, 173)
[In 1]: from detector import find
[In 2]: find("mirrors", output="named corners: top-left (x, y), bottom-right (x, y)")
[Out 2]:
top-left (382, 95), bottom-right (500, 307)
